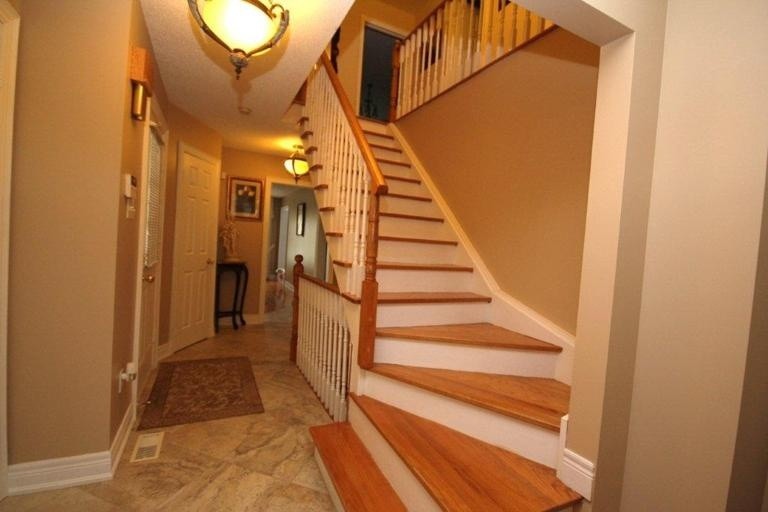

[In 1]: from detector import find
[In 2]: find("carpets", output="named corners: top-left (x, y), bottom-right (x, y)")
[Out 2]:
top-left (135, 355), bottom-right (265, 431)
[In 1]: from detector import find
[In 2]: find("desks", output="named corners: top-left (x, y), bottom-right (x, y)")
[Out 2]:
top-left (214, 260), bottom-right (249, 334)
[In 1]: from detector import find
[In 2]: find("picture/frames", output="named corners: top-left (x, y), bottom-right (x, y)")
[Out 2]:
top-left (296, 203), bottom-right (305, 236)
top-left (225, 175), bottom-right (264, 222)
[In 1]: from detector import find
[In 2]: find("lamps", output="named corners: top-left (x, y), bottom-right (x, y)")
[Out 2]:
top-left (187, 0), bottom-right (289, 82)
top-left (283, 145), bottom-right (309, 184)
